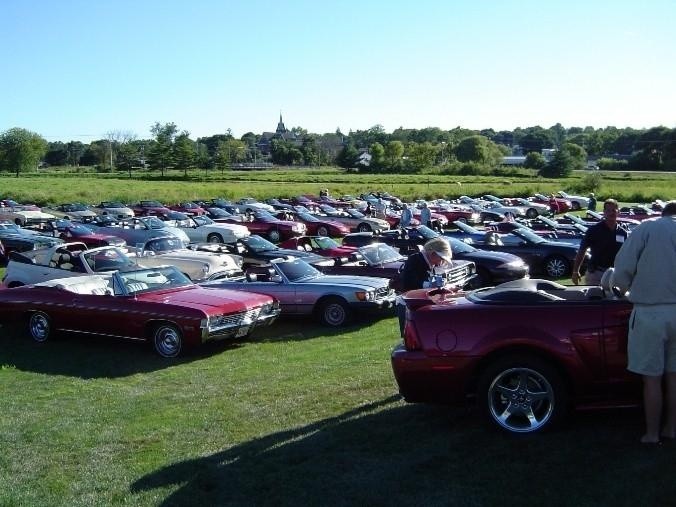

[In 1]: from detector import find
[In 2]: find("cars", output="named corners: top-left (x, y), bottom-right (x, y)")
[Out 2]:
top-left (391, 268), bottom-right (644, 436)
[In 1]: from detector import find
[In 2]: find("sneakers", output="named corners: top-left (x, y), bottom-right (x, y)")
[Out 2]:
top-left (630, 434), bottom-right (676, 451)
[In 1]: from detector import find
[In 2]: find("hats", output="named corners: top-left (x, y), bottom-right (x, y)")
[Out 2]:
top-left (424, 239), bottom-right (453, 264)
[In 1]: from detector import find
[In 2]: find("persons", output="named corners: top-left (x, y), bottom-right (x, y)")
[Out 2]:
top-left (572, 199), bottom-right (628, 286)
top-left (389, 238), bottom-right (454, 345)
top-left (320, 189), bottom-right (328, 196)
top-left (367, 198), bottom-right (386, 220)
top-left (548, 194), bottom-right (559, 219)
top-left (400, 203), bottom-right (413, 227)
top-left (589, 193), bottom-right (596, 212)
top-left (421, 203), bottom-right (431, 225)
top-left (612, 202), bottom-right (676, 443)
